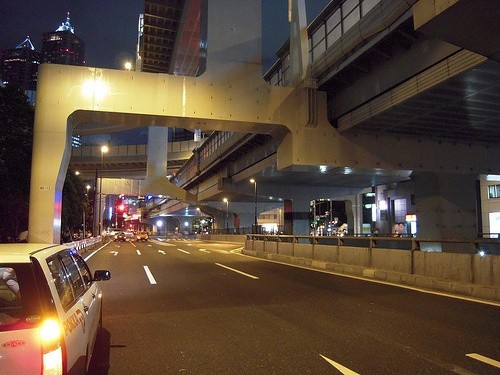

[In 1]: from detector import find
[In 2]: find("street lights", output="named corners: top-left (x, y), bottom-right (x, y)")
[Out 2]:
top-left (250, 178), bottom-right (258, 234)
top-left (223, 198), bottom-right (228, 234)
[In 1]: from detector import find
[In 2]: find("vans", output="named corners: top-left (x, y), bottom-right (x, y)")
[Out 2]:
top-left (0, 243), bottom-right (111, 375)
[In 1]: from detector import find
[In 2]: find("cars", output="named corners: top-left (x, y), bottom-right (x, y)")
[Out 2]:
top-left (108, 227), bottom-right (149, 242)
